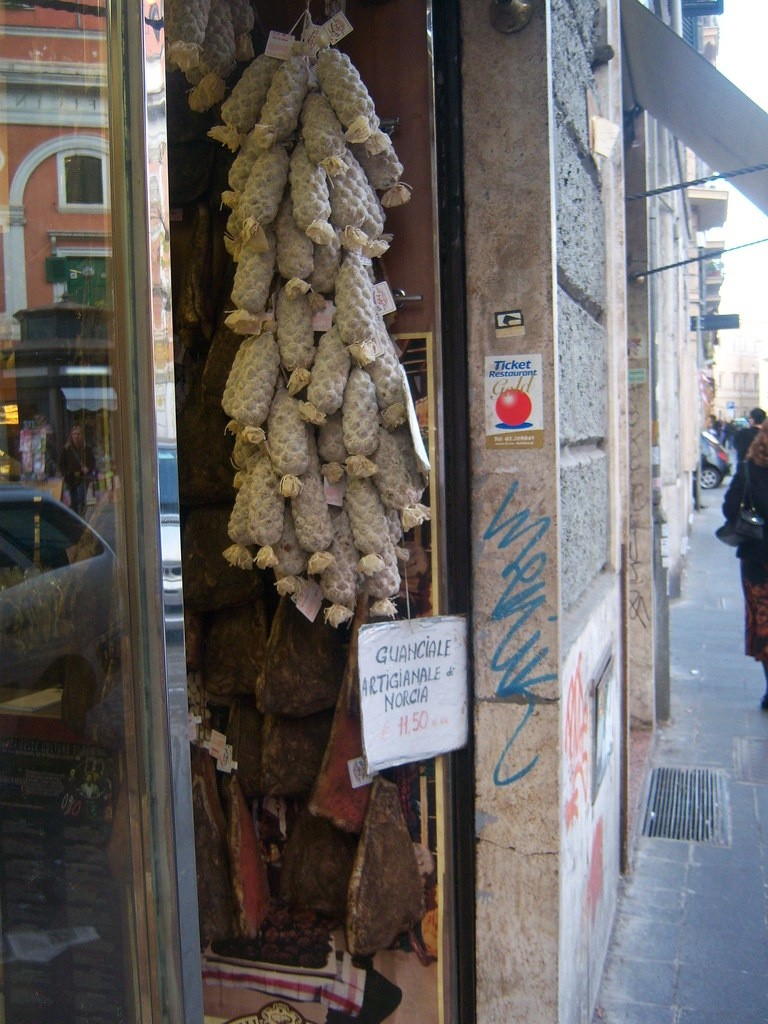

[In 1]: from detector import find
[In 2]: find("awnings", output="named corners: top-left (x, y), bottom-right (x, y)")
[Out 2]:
top-left (60, 386), bottom-right (118, 412)
top-left (620, 0), bottom-right (768, 279)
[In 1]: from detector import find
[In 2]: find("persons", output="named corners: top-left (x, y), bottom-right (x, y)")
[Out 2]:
top-left (57, 426), bottom-right (96, 514)
top-left (734, 410), bottom-right (765, 462)
top-left (722, 418), bottom-right (768, 710)
top-left (57, 490), bottom-right (121, 730)
top-left (710, 414), bottom-right (736, 449)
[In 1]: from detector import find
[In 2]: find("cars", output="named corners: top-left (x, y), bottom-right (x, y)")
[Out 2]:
top-left (0, 485), bottom-right (121, 729)
top-left (152, 435), bottom-right (185, 634)
top-left (698, 431), bottom-right (738, 490)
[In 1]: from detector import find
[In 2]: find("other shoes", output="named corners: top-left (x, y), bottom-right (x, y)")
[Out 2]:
top-left (761, 694), bottom-right (768, 708)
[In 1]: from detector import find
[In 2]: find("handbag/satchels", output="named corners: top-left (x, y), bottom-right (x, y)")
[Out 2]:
top-left (715, 460), bottom-right (764, 547)
